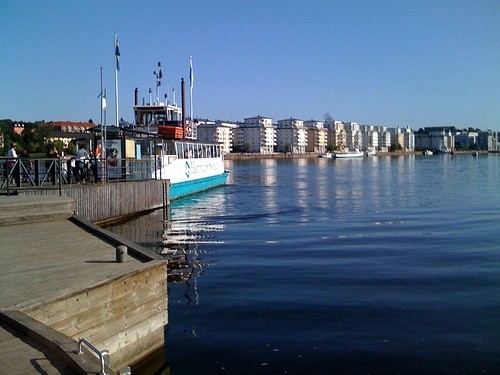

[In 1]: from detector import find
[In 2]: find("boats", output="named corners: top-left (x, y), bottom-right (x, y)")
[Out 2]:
top-left (87, 33), bottom-right (230, 204)
top-left (321, 147), bottom-right (364, 158)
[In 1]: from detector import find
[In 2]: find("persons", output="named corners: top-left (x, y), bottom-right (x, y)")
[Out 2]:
top-left (6, 142), bottom-right (17, 168)
top-left (74, 145), bottom-right (91, 184)
top-left (19, 150), bottom-right (32, 181)
top-left (46, 147), bottom-right (60, 168)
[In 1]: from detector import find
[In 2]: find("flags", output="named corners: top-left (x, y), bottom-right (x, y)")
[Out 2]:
top-left (190, 66), bottom-right (195, 88)
top-left (114, 35), bottom-right (121, 72)
